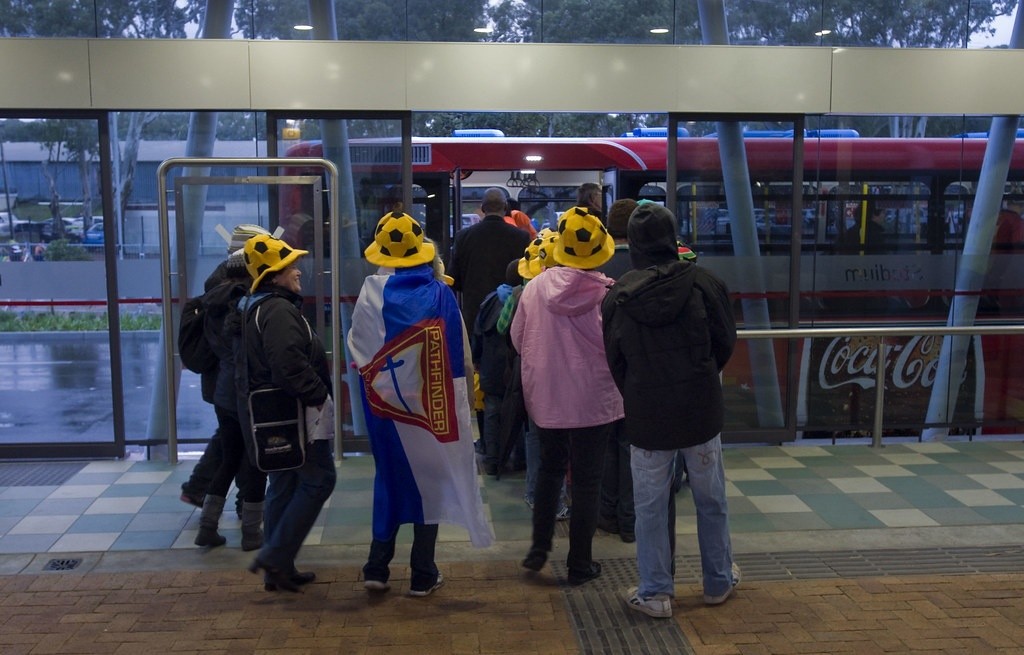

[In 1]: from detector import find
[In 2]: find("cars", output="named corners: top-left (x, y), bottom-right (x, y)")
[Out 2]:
top-left (0, 213), bottom-right (109, 250)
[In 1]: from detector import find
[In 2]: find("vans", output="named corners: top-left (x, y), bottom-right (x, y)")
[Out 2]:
top-left (448, 213), bottom-right (480, 250)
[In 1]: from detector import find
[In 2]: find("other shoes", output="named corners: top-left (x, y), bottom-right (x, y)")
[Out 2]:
top-left (362, 579), bottom-right (390, 590)
top-left (523, 492), bottom-right (534, 509)
top-left (409, 568), bottom-right (443, 596)
top-left (556, 503), bottom-right (571, 520)
top-left (520, 555), bottom-right (546, 570)
top-left (621, 530), bottom-right (636, 543)
top-left (180, 489), bottom-right (204, 508)
top-left (567, 561), bottom-right (601, 587)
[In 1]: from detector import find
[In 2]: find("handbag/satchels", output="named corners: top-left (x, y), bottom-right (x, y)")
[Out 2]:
top-left (237, 385), bottom-right (306, 472)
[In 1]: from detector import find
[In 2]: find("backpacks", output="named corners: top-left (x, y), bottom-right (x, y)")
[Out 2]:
top-left (177, 280), bottom-right (237, 374)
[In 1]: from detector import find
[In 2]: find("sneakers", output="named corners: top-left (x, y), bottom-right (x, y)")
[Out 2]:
top-left (626, 586), bottom-right (672, 618)
top-left (702, 562), bottom-right (741, 604)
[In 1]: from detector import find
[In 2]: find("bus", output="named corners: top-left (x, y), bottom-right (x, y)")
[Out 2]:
top-left (278, 128), bottom-right (1023, 440)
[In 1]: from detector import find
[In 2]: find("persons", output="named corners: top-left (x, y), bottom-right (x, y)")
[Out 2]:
top-left (994, 191), bottom-right (1024, 242)
top-left (347, 182), bottom-right (743, 615)
top-left (845, 202), bottom-right (886, 254)
top-left (181, 224), bottom-right (337, 593)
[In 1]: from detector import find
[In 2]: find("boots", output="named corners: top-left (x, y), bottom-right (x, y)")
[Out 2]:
top-left (194, 494), bottom-right (227, 547)
top-left (240, 498), bottom-right (267, 551)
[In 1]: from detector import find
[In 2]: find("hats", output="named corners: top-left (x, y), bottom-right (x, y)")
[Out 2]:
top-left (365, 211), bottom-right (435, 267)
top-left (553, 206), bottom-right (615, 269)
top-left (242, 234), bottom-right (309, 295)
top-left (228, 223), bottom-right (270, 255)
top-left (518, 227), bottom-right (561, 279)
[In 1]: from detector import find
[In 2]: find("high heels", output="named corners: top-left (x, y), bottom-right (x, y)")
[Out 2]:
top-left (248, 556), bottom-right (299, 592)
top-left (265, 569), bottom-right (316, 591)
